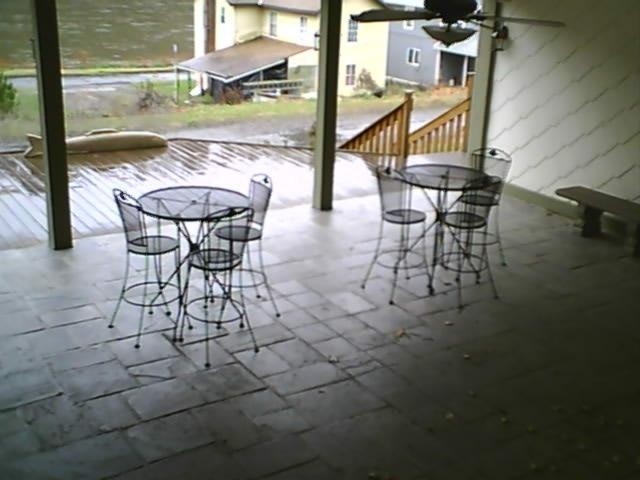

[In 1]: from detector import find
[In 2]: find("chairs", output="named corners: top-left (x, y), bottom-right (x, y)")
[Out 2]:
top-left (356, 146), bottom-right (512, 310)
top-left (110, 174), bottom-right (279, 367)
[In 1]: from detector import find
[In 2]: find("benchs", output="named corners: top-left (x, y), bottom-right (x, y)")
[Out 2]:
top-left (555, 186), bottom-right (640, 256)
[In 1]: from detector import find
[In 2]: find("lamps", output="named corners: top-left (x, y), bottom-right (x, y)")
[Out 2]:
top-left (490, 25), bottom-right (508, 51)
top-left (421, 20), bottom-right (478, 48)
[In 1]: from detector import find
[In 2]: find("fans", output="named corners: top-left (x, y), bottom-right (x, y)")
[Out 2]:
top-left (350, 0), bottom-right (564, 48)
